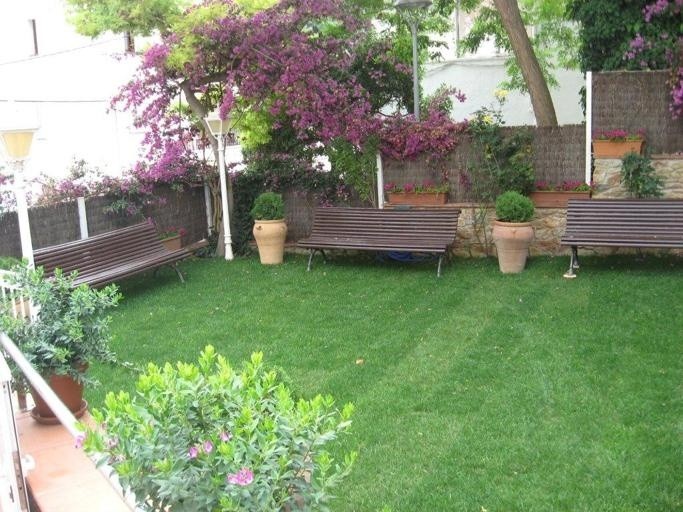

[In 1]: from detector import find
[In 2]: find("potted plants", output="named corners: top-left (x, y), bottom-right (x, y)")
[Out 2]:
top-left (0, 254), bottom-right (148, 425)
top-left (250, 192), bottom-right (289, 265)
top-left (491, 190), bottom-right (534, 275)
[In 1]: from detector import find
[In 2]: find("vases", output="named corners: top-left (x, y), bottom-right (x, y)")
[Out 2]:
top-left (160, 235), bottom-right (182, 252)
top-left (531, 189), bottom-right (591, 208)
top-left (385, 191), bottom-right (449, 206)
top-left (590, 139), bottom-right (648, 159)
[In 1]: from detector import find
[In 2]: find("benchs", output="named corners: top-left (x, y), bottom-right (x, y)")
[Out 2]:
top-left (559, 198), bottom-right (683, 278)
top-left (34, 221), bottom-right (209, 297)
top-left (297, 206), bottom-right (462, 276)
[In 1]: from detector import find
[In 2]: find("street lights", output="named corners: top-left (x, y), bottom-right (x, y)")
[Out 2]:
top-left (203, 104), bottom-right (238, 260)
top-left (393, 0), bottom-right (433, 122)
top-left (0, 113), bottom-right (40, 325)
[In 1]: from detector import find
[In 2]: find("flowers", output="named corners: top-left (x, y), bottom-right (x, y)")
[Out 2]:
top-left (591, 127), bottom-right (649, 143)
top-left (383, 180), bottom-right (452, 194)
top-left (72, 344), bottom-right (362, 512)
top-left (534, 179), bottom-right (599, 193)
top-left (146, 215), bottom-right (186, 239)
